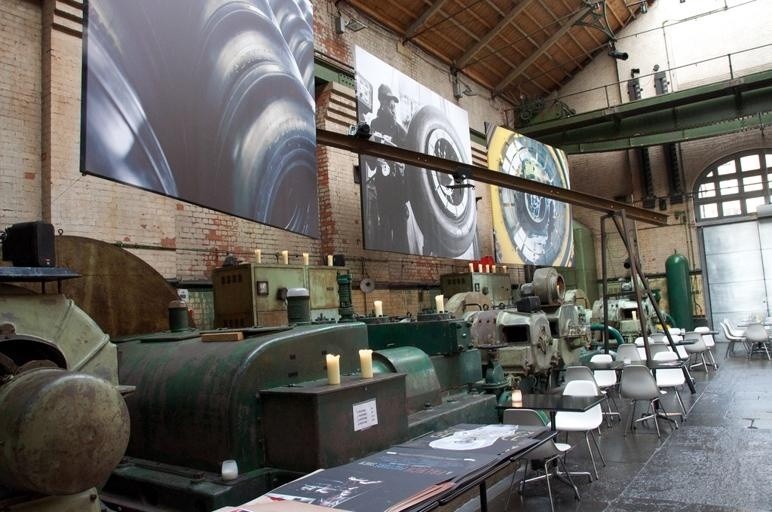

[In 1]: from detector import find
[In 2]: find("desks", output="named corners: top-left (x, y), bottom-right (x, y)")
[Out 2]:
top-left (238, 423), bottom-right (560, 512)
top-left (503, 394), bottom-right (607, 411)
top-left (586, 359), bottom-right (689, 370)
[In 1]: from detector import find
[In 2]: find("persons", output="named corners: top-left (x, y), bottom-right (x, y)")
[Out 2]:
top-left (366, 82), bottom-right (418, 256)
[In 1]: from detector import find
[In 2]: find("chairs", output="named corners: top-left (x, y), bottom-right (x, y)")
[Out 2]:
top-left (648, 351), bottom-right (688, 421)
top-left (555, 379), bottom-right (608, 479)
top-left (615, 320), bottom-right (771, 382)
top-left (566, 365), bottom-right (612, 429)
top-left (503, 409), bottom-right (581, 511)
top-left (587, 353), bottom-right (621, 423)
top-left (619, 364), bottom-right (667, 439)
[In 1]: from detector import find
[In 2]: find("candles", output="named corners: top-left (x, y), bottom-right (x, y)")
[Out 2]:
top-left (468, 262), bottom-right (507, 273)
top-left (255, 249), bottom-right (261, 265)
top-left (282, 250), bottom-right (289, 265)
top-left (328, 255), bottom-right (334, 267)
top-left (303, 253), bottom-right (309, 266)
top-left (435, 295), bottom-right (444, 314)
top-left (374, 301), bottom-right (383, 318)
top-left (358, 349), bottom-right (374, 378)
top-left (325, 354), bottom-right (341, 385)
